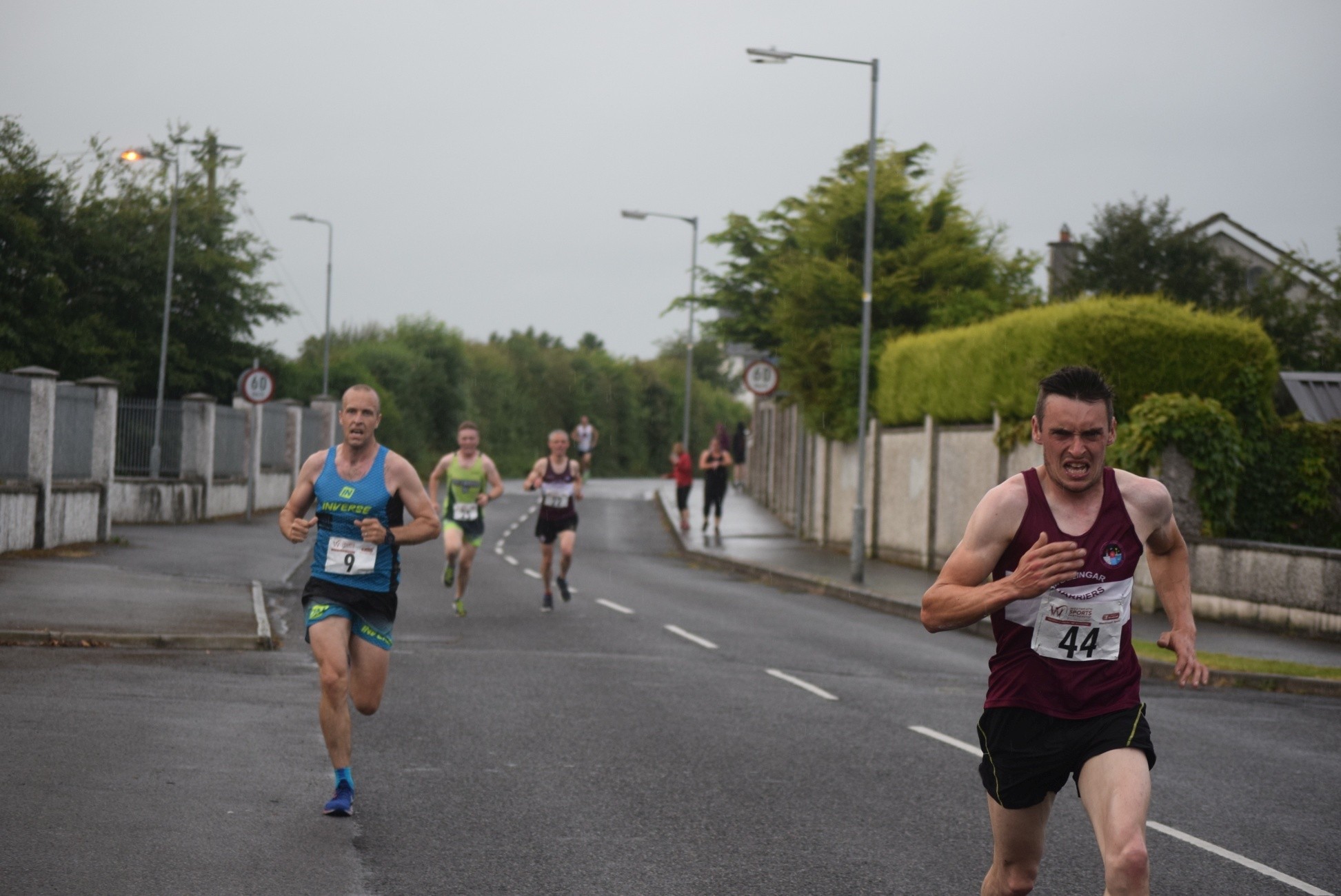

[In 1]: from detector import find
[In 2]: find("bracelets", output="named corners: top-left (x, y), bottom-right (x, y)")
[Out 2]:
top-left (488, 494), bottom-right (492, 502)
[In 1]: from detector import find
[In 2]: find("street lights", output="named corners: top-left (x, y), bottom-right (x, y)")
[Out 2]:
top-left (122, 150), bottom-right (181, 480)
top-left (743, 45), bottom-right (881, 586)
top-left (289, 213), bottom-right (335, 396)
top-left (620, 208), bottom-right (700, 454)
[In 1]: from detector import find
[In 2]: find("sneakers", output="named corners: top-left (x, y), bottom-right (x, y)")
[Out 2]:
top-left (322, 778), bottom-right (356, 817)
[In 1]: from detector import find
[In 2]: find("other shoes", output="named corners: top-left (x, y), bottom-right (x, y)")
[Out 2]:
top-left (452, 597), bottom-right (465, 617)
top-left (556, 576), bottom-right (570, 602)
top-left (540, 592), bottom-right (554, 613)
top-left (441, 564), bottom-right (455, 587)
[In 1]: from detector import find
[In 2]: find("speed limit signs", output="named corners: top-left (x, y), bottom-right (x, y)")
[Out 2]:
top-left (244, 370), bottom-right (273, 405)
top-left (743, 360), bottom-right (778, 396)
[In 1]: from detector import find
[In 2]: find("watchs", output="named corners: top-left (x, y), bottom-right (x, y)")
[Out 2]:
top-left (384, 526), bottom-right (395, 546)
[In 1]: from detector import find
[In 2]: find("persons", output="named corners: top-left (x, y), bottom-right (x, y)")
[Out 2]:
top-left (280, 384), bottom-right (442, 814)
top-left (697, 439), bottom-right (734, 535)
top-left (920, 366), bottom-right (1208, 896)
top-left (732, 418), bottom-right (745, 488)
top-left (524, 430), bottom-right (584, 613)
top-left (571, 416), bottom-right (599, 478)
top-left (660, 442), bottom-right (693, 533)
top-left (430, 421), bottom-right (504, 619)
